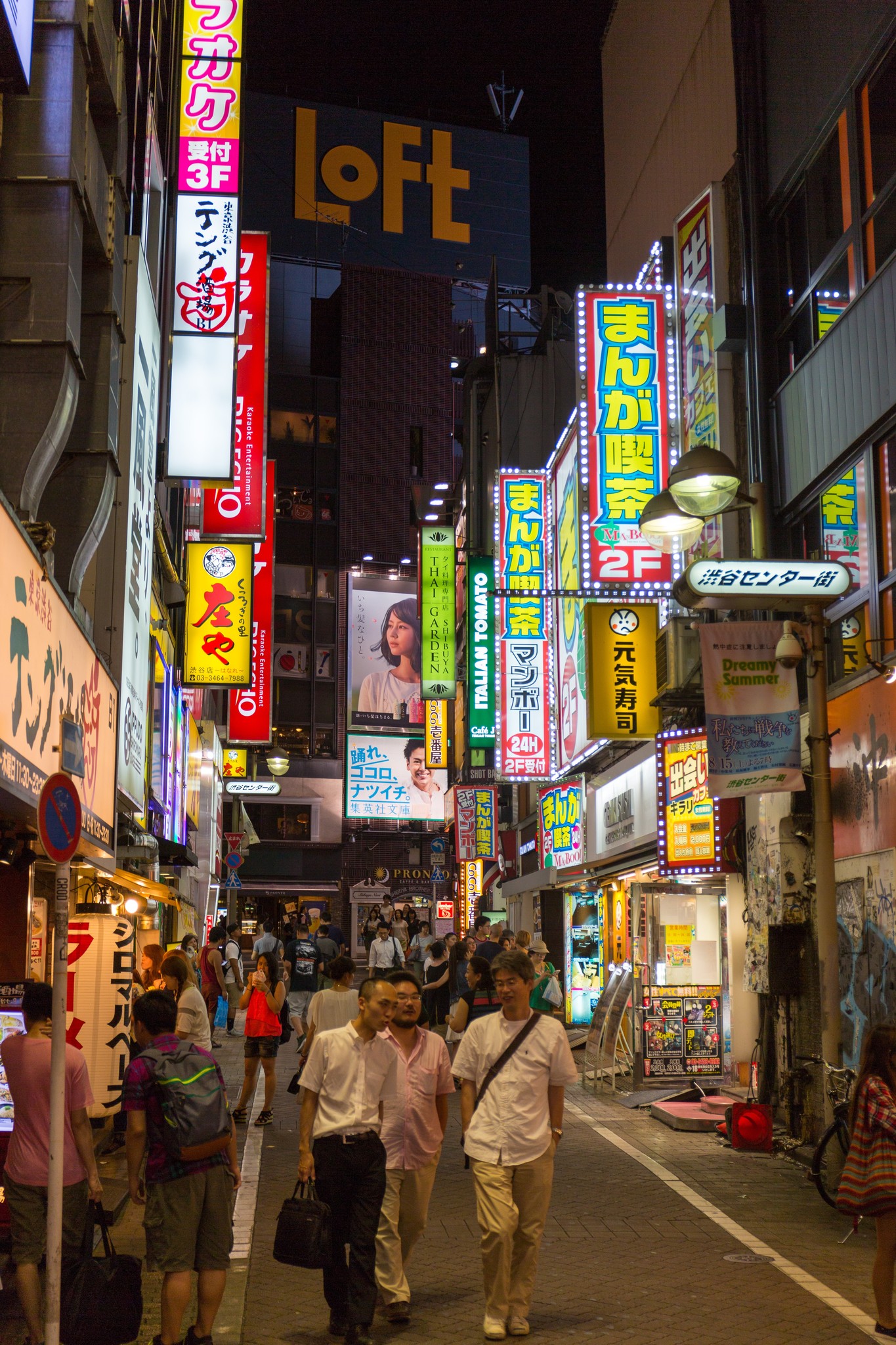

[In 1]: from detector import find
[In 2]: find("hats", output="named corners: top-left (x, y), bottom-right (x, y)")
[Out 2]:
top-left (528, 940), bottom-right (549, 953)
top-left (227, 922), bottom-right (239, 934)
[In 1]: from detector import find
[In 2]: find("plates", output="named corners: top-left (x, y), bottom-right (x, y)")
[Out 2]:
top-left (0, 1016), bottom-right (26, 1122)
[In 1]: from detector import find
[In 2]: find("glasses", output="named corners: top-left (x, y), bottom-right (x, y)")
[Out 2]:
top-left (503, 944), bottom-right (511, 948)
top-left (532, 951), bottom-right (548, 956)
top-left (467, 942), bottom-right (477, 945)
top-left (378, 931), bottom-right (388, 934)
top-left (480, 925), bottom-right (490, 929)
top-left (396, 994), bottom-right (423, 1001)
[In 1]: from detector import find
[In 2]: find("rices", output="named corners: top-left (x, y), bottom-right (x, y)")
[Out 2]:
top-left (0, 1106), bottom-right (15, 1119)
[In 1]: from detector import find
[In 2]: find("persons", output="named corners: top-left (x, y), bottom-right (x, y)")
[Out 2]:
top-left (403, 739), bottom-right (446, 819)
top-left (230, 952), bottom-right (286, 1126)
top-left (357, 598), bottom-right (425, 712)
top-left (446, 955), bottom-right (503, 1034)
top-left (368, 921), bottom-right (405, 978)
top-left (528, 940), bottom-right (562, 1017)
top-left (0, 896), bottom-right (578, 1345)
top-left (160, 956), bottom-right (212, 1052)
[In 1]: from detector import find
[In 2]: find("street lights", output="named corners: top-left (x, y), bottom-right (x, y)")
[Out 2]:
top-left (641, 446), bottom-right (772, 563)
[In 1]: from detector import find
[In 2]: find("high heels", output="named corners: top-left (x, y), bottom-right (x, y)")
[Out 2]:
top-left (231, 1108), bottom-right (247, 1123)
top-left (254, 1108), bottom-right (274, 1125)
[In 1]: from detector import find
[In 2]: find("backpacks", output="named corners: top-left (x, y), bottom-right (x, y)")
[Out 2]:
top-left (133, 1041), bottom-right (234, 1160)
top-left (219, 940), bottom-right (242, 977)
top-left (251, 979), bottom-right (295, 1044)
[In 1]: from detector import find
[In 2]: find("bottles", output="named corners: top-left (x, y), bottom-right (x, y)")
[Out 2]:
top-left (242, 912), bottom-right (257, 919)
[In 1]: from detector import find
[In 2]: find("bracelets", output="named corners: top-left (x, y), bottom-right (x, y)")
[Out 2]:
top-left (265, 990), bottom-right (271, 997)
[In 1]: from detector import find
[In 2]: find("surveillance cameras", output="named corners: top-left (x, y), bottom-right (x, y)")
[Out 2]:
top-left (774, 634), bottom-right (803, 670)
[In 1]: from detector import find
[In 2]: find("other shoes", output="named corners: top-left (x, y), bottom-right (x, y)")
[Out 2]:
top-left (101, 1132), bottom-right (125, 1155)
top-left (875, 1321), bottom-right (896, 1340)
top-left (153, 1334), bottom-right (161, 1345)
top-left (211, 1040), bottom-right (222, 1048)
top-left (347, 1325), bottom-right (377, 1345)
top-left (387, 1301), bottom-right (410, 1320)
top-left (326, 1322), bottom-right (346, 1335)
top-left (179, 1326), bottom-right (213, 1345)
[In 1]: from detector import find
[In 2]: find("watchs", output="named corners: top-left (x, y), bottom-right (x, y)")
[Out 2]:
top-left (551, 1127), bottom-right (564, 1139)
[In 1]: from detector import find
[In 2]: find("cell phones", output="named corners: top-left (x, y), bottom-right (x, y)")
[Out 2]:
top-left (553, 970), bottom-right (560, 974)
top-left (222, 961), bottom-right (228, 966)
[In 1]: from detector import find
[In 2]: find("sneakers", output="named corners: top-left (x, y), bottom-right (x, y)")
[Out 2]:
top-left (483, 1310), bottom-right (506, 1339)
top-left (508, 1315), bottom-right (529, 1335)
top-left (226, 1028), bottom-right (244, 1037)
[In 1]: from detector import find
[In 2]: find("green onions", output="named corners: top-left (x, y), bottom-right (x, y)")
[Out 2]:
top-left (5, 1106), bottom-right (11, 1110)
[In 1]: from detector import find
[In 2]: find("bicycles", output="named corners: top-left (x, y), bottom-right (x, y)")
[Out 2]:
top-left (793, 1053), bottom-right (863, 1217)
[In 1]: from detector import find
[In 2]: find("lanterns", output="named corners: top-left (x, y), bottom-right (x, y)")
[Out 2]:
top-left (64, 901), bottom-right (134, 1134)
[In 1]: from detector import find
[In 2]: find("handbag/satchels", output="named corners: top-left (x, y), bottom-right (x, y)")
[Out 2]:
top-left (287, 1062), bottom-right (306, 1095)
top-left (542, 976), bottom-right (563, 1008)
top-left (408, 935), bottom-right (420, 961)
top-left (393, 937), bottom-right (402, 967)
top-left (214, 996), bottom-right (228, 1028)
top-left (273, 1178), bottom-right (330, 1270)
top-left (62, 1198), bottom-right (142, 1345)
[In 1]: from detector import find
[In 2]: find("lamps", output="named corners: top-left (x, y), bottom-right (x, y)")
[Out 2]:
top-left (106, 885), bottom-right (124, 905)
top-left (608, 959), bottom-right (617, 971)
top-left (615, 967), bottom-right (623, 976)
top-left (628, 962), bottom-right (632, 972)
top-left (622, 958), bottom-right (630, 969)
